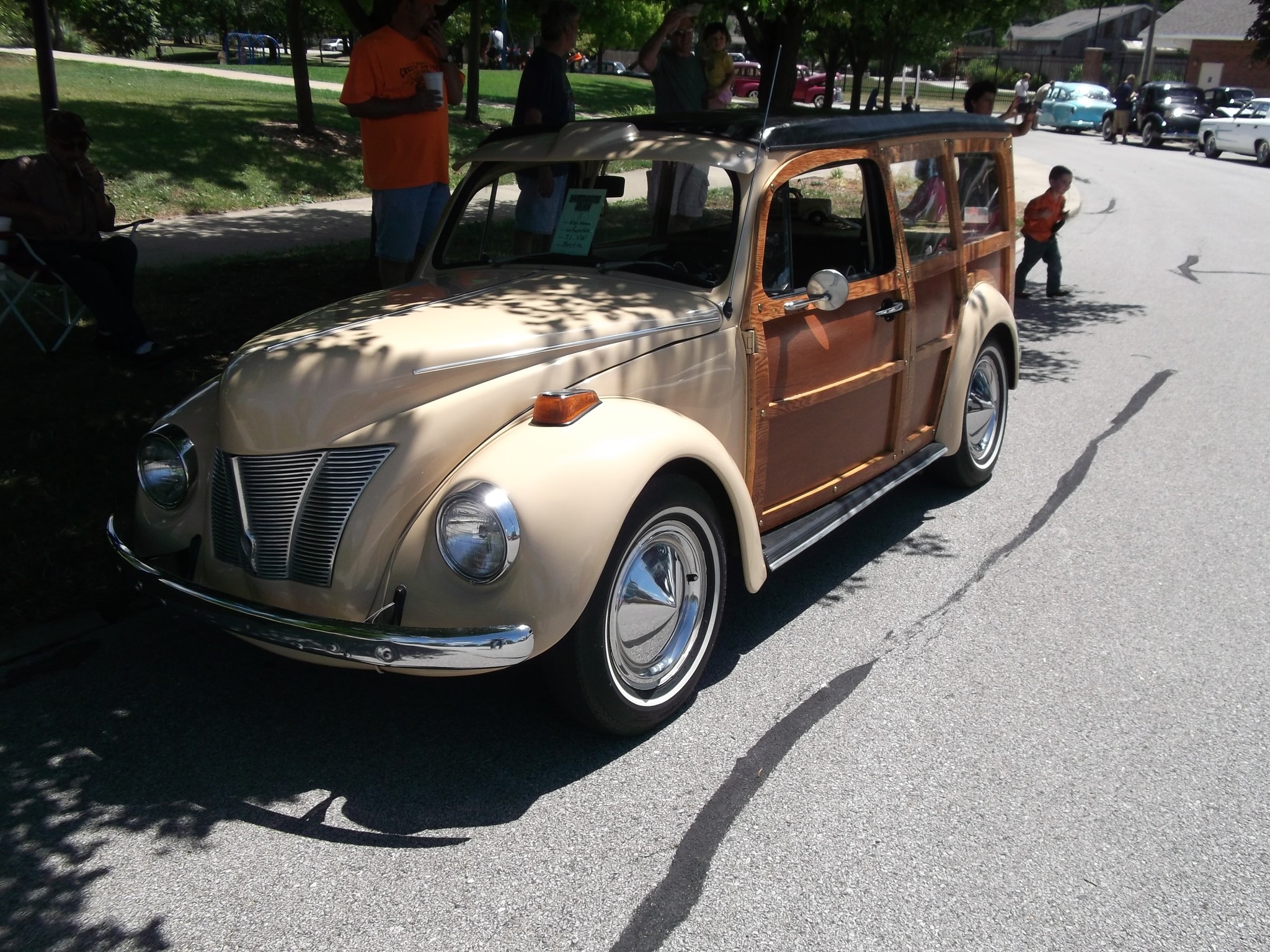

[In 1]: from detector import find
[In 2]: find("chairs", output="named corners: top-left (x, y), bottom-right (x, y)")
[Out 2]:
top-left (0, 159), bottom-right (153, 352)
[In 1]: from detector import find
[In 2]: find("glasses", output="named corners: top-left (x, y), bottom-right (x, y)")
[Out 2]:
top-left (57, 142), bottom-right (90, 152)
top-left (673, 28), bottom-right (694, 36)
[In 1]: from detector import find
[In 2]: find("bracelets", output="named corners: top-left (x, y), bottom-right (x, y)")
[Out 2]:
top-left (1024, 117), bottom-right (1033, 122)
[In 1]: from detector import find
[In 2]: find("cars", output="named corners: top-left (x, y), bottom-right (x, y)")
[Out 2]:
top-left (727, 51), bottom-right (842, 110)
top-left (1196, 85), bottom-right (1270, 167)
top-left (321, 37), bottom-right (350, 52)
top-left (95, 100), bottom-right (1022, 743)
top-left (1031, 80), bottom-right (1117, 134)
top-left (582, 60), bottom-right (651, 80)
top-left (1100, 81), bottom-right (1218, 149)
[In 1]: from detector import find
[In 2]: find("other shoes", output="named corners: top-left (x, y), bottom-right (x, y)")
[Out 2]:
top-left (93, 331), bottom-right (129, 367)
top-left (1015, 288), bottom-right (1031, 298)
top-left (1046, 287), bottom-right (1069, 297)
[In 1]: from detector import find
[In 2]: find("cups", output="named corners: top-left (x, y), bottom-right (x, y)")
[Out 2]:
top-left (423, 72), bottom-right (443, 104)
top-left (0, 216), bottom-right (11, 255)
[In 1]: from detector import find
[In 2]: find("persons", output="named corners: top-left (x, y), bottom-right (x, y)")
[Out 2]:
top-left (0, 114), bottom-right (164, 376)
top-left (901, 155), bottom-right (948, 252)
top-left (567, 52), bottom-right (589, 69)
top-left (865, 88), bottom-right (881, 111)
top-left (901, 95), bottom-right (915, 113)
top-left (1014, 73), bottom-right (1055, 129)
top-left (510, 3), bottom-right (580, 256)
top-left (483, 27), bottom-right (532, 70)
top-left (340, 0), bottom-right (467, 289)
top-left (636, 7), bottom-right (735, 235)
top-left (960, 79), bottom-right (1037, 220)
top-left (1015, 165), bottom-right (1073, 298)
top-left (1112, 74), bottom-right (1149, 144)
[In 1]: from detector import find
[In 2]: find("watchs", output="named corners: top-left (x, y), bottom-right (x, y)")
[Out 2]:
top-left (441, 54), bottom-right (453, 63)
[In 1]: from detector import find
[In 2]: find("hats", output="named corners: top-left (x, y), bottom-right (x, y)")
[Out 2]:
top-left (45, 111), bottom-right (93, 142)
